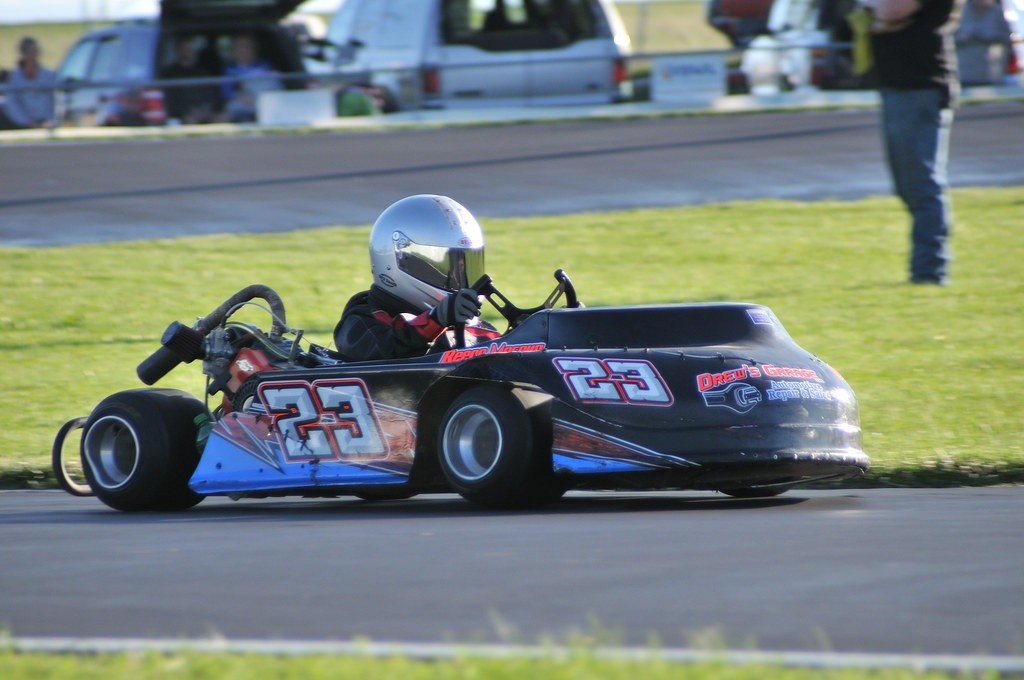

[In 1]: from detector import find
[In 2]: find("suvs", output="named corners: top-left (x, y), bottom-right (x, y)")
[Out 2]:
top-left (51, 0), bottom-right (322, 127)
top-left (305, 0), bottom-right (638, 113)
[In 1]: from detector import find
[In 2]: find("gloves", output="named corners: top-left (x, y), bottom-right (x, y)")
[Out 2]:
top-left (427, 289), bottom-right (480, 324)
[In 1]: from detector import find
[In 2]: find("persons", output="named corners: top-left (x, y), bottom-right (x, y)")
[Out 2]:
top-left (161, 32), bottom-right (279, 126)
top-left (4, 37), bottom-right (60, 129)
top-left (869, 1), bottom-right (964, 285)
top-left (333, 194), bottom-right (503, 362)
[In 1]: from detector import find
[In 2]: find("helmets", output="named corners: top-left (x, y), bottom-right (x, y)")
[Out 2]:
top-left (370, 194), bottom-right (486, 314)
